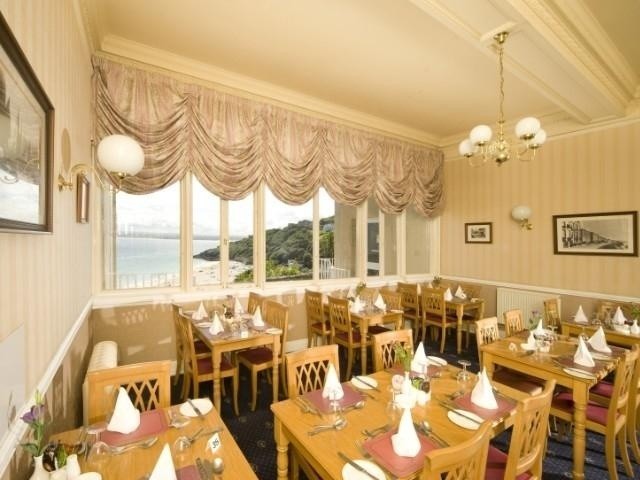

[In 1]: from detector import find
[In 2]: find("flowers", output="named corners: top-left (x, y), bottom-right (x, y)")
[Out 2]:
top-left (18, 390), bottom-right (53, 457)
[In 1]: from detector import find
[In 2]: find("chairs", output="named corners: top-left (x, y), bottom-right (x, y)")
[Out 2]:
top-left (86, 360), bottom-right (171, 424)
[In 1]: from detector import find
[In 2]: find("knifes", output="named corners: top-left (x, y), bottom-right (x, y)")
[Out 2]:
top-left (338, 452), bottom-right (380, 480)
top-left (414, 423), bottom-right (444, 448)
top-left (352, 375), bottom-right (381, 393)
top-left (438, 402), bottom-right (483, 425)
top-left (552, 359), bottom-right (596, 378)
top-left (186, 397), bottom-right (204, 421)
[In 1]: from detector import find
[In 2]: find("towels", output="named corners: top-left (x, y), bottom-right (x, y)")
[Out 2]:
top-left (106, 387), bottom-right (141, 434)
top-left (148, 442), bottom-right (178, 480)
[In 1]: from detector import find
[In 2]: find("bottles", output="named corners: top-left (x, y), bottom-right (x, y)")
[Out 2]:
top-left (411, 377), bottom-right (430, 393)
top-left (48, 439), bottom-right (69, 468)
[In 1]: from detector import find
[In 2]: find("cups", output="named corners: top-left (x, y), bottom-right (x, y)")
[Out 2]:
top-left (392, 374), bottom-right (405, 389)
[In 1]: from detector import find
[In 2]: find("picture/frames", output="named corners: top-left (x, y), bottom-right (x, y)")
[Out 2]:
top-left (0, 12), bottom-right (56, 236)
top-left (552, 211), bottom-right (637, 257)
top-left (465, 222), bottom-right (492, 244)
top-left (77, 172), bottom-right (90, 223)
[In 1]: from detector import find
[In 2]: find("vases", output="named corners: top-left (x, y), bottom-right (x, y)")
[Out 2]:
top-left (29, 454), bottom-right (49, 480)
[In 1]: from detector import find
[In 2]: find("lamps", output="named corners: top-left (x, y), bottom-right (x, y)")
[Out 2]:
top-left (58, 132), bottom-right (145, 193)
top-left (512, 204), bottom-right (533, 230)
top-left (458, 30), bottom-right (550, 165)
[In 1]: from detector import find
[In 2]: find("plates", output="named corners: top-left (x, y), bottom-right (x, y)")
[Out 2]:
top-left (180, 399), bottom-right (213, 416)
top-left (564, 368), bottom-right (596, 380)
top-left (341, 460), bottom-right (387, 480)
top-left (530, 329), bottom-right (552, 338)
top-left (74, 472), bottom-right (102, 480)
top-left (447, 409), bottom-right (484, 430)
top-left (351, 376), bottom-right (377, 389)
top-left (589, 352), bottom-right (611, 361)
top-left (426, 355), bottom-right (447, 367)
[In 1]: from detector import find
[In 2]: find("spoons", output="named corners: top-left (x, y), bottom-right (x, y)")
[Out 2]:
top-left (212, 458), bottom-right (224, 480)
top-left (112, 436), bottom-right (159, 454)
top-left (168, 409), bottom-right (175, 428)
top-left (422, 418), bottom-right (451, 447)
top-left (491, 384), bottom-right (523, 404)
top-left (342, 400), bottom-right (367, 415)
top-left (308, 418), bottom-right (348, 436)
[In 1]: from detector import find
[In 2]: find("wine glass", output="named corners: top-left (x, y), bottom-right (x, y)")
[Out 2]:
top-left (172, 418), bottom-right (195, 469)
top-left (457, 359), bottom-right (471, 386)
top-left (387, 386), bottom-right (401, 431)
top-left (86, 423), bottom-right (111, 466)
top-left (592, 305), bottom-right (612, 330)
top-left (577, 322), bottom-right (589, 344)
top-left (537, 326), bottom-right (559, 362)
